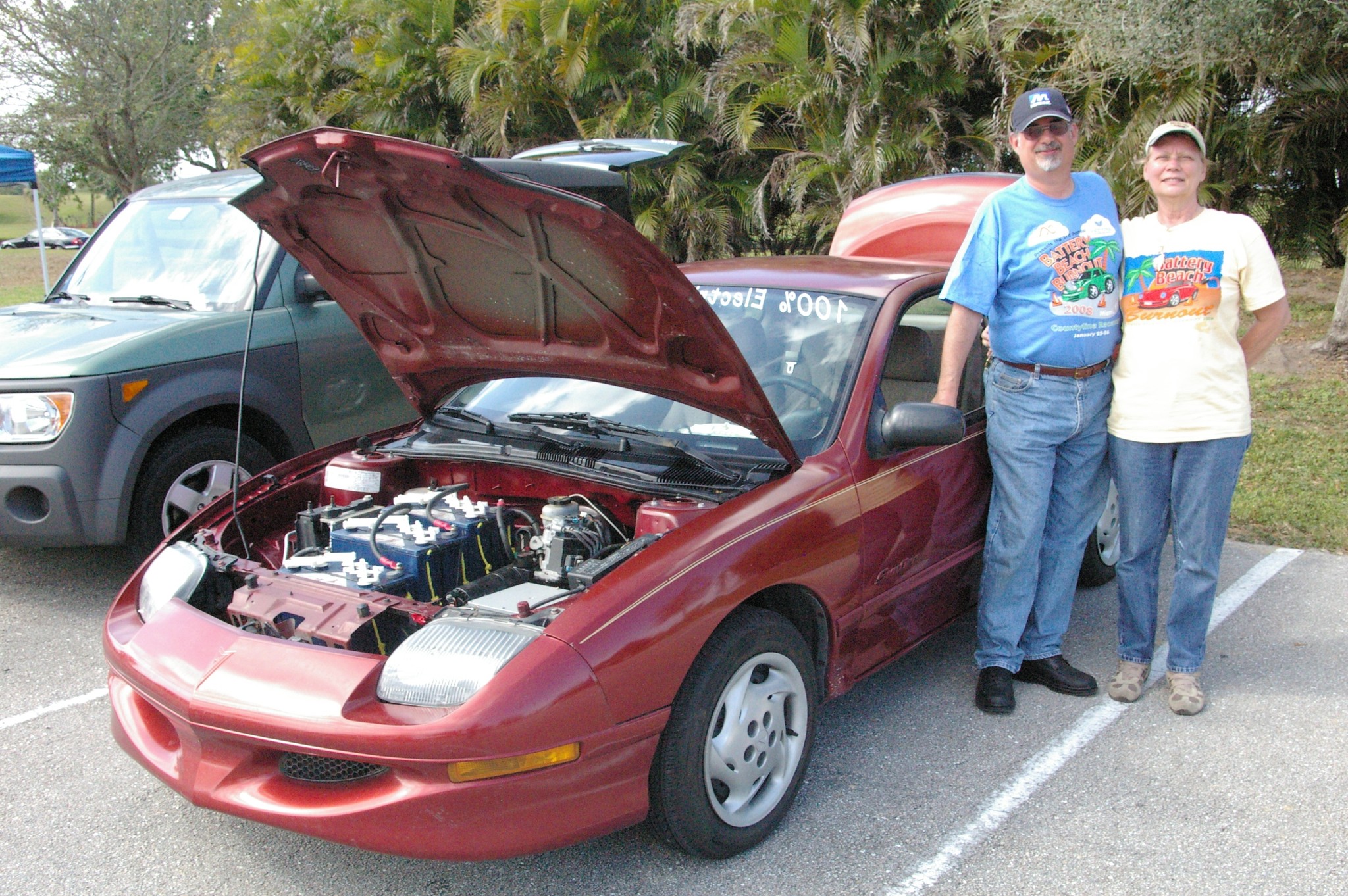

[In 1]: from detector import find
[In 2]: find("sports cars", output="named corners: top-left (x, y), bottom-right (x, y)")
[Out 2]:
top-left (94, 123), bottom-right (1026, 870)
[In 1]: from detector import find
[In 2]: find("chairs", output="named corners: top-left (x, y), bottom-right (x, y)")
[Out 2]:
top-left (697, 315), bottom-right (787, 424)
top-left (880, 322), bottom-right (939, 411)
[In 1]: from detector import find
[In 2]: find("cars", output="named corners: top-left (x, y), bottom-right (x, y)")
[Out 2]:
top-left (0, 225), bottom-right (92, 249)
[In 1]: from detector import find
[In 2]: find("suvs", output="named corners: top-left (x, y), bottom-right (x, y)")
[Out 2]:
top-left (0, 133), bottom-right (694, 566)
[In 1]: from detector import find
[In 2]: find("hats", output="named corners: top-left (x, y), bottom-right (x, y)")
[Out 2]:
top-left (1145, 120), bottom-right (1206, 159)
top-left (1009, 88), bottom-right (1073, 133)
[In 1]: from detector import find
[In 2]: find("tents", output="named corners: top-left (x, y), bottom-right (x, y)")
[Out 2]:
top-left (0, 145), bottom-right (50, 297)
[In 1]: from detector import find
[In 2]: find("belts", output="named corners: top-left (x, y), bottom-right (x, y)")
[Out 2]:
top-left (1000, 357), bottom-right (1111, 379)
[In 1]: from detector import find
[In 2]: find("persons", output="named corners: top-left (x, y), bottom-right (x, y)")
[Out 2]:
top-left (931, 87), bottom-right (1124, 715)
top-left (983, 120), bottom-right (1291, 716)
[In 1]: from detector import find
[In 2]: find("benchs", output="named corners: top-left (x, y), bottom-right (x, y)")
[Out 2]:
top-left (784, 317), bottom-right (987, 417)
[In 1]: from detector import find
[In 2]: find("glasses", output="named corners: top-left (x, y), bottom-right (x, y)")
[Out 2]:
top-left (1020, 122), bottom-right (1074, 140)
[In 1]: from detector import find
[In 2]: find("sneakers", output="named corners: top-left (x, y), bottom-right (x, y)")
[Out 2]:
top-left (975, 663), bottom-right (1016, 713)
top-left (1017, 654), bottom-right (1097, 696)
top-left (1109, 659), bottom-right (1150, 704)
top-left (1166, 668), bottom-right (1205, 715)
